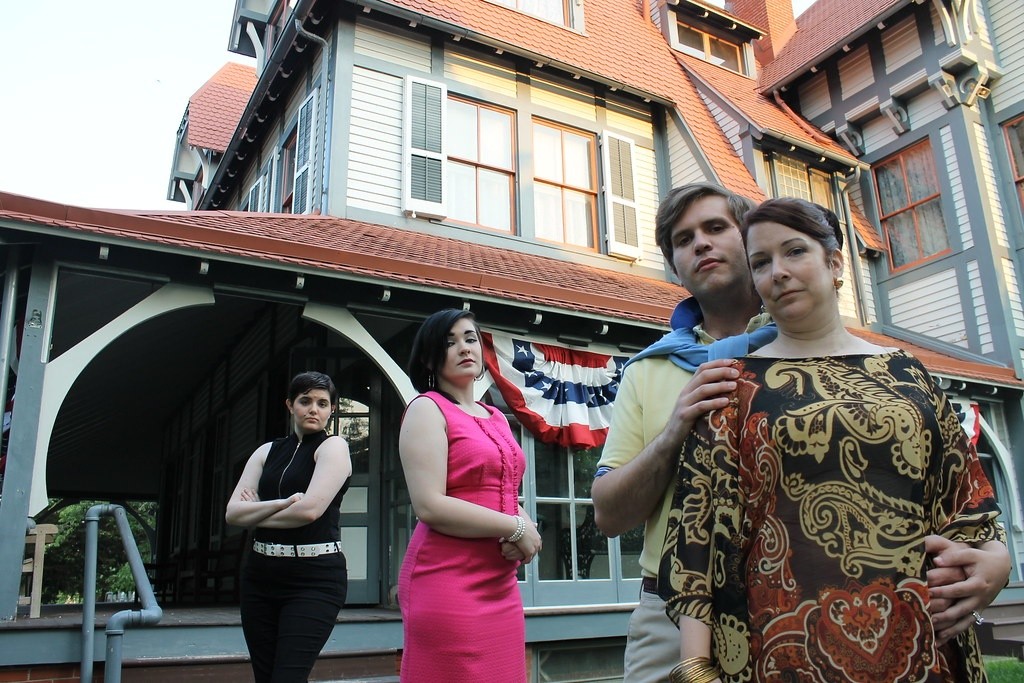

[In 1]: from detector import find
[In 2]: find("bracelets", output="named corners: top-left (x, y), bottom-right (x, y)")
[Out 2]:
top-left (669, 656), bottom-right (720, 683)
top-left (507, 516), bottom-right (525, 542)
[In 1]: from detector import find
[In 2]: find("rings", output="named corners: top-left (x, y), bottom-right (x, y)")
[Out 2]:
top-left (973, 611), bottom-right (983, 625)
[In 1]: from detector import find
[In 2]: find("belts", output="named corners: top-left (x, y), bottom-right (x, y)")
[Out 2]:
top-left (642, 576), bottom-right (659, 594)
top-left (252, 540), bottom-right (341, 557)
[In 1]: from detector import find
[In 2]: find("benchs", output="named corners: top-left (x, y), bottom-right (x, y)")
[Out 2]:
top-left (132, 527), bottom-right (248, 601)
top-left (558, 487), bottom-right (645, 579)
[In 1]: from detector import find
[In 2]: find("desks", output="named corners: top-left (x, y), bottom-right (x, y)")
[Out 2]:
top-left (18, 523), bottom-right (59, 619)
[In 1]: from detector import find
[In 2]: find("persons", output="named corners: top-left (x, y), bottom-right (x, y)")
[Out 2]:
top-left (657, 195), bottom-right (1011, 683)
top-left (397, 309), bottom-right (542, 683)
top-left (225, 372), bottom-right (353, 683)
top-left (593, 182), bottom-right (968, 683)
